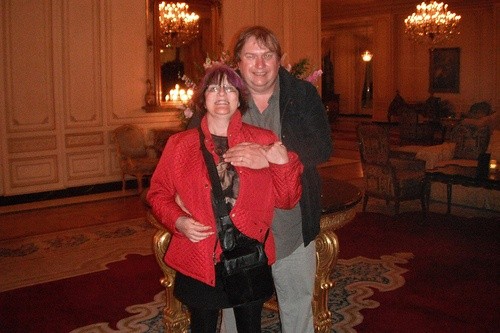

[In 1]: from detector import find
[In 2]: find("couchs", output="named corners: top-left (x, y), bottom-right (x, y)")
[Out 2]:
top-left (461, 111), bottom-right (500, 131)
top-left (415, 129), bottom-right (500, 211)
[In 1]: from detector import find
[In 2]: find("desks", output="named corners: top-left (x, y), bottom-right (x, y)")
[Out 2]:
top-left (153, 124), bottom-right (185, 159)
top-left (142, 179), bottom-right (365, 332)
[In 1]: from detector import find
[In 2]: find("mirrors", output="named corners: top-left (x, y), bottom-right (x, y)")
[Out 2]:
top-left (153, 0), bottom-right (224, 110)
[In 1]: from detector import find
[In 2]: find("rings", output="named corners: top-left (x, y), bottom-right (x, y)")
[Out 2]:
top-left (239, 156), bottom-right (243, 162)
top-left (280, 144), bottom-right (284, 146)
top-left (194, 221), bottom-right (197, 224)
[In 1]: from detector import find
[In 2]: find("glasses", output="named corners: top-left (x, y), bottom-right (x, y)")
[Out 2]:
top-left (206, 85), bottom-right (239, 94)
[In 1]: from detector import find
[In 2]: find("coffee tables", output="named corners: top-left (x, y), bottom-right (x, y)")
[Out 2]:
top-left (439, 117), bottom-right (462, 143)
top-left (421, 165), bottom-right (500, 217)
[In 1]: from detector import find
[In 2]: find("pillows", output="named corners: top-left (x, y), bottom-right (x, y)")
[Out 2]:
top-left (452, 125), bottom-right (489, 159)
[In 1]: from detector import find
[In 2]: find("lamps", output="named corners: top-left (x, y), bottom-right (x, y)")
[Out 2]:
top-left (158, 2), bottom-right (201, 47)
top-left (404, 0), bottom-right (462, 45)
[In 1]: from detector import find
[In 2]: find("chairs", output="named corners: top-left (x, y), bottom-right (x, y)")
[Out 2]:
top-left (113, 126), bottom-right (158, 193)
top-left (356, 99), bottom-right (490, 219)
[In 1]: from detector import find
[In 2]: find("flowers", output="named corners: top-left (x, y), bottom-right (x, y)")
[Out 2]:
top-left (288, 59), bottom-right (324, 89)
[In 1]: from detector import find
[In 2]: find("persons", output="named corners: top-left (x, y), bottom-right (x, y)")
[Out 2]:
top-left (181, 25), bottom-right (333, 333)
top-left (145, 65), bottom-right (304, 333)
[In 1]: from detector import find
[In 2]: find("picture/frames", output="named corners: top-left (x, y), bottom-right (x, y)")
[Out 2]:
top-left (430, 47), bottom-right (462, 93)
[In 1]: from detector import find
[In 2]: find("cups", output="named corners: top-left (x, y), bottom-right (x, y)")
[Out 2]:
top-left (489, 160), bottom-right (497, 174)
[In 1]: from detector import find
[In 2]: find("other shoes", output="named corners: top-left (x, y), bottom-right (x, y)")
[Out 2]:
top-left (221, 239), bottom-right (266, 278)
